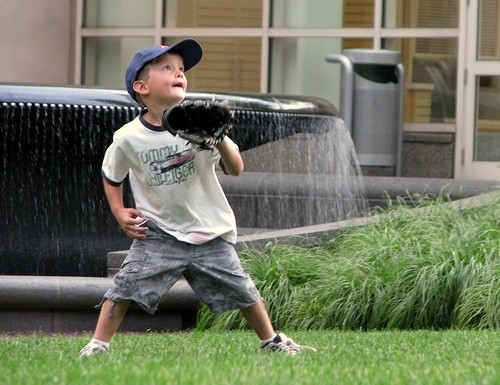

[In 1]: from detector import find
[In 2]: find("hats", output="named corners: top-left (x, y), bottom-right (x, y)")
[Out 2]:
top-left (126, 38), bottom-right (202, 103)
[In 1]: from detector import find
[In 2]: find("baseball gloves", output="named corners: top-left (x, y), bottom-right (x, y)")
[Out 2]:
top-left (161, 100), bottom-right (236, 146)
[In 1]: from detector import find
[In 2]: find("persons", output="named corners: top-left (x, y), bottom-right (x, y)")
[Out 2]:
top-left (79, 39), bottom-right (318, 357)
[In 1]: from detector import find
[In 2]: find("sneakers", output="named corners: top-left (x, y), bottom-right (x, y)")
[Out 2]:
top-left (79, 340), bottom-right (110, 356)
top-left (260, 332), bottom-right (317, 356)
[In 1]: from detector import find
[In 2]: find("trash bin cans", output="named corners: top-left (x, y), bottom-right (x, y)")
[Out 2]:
top-left (340, 49), bottom-right (404, 166)
